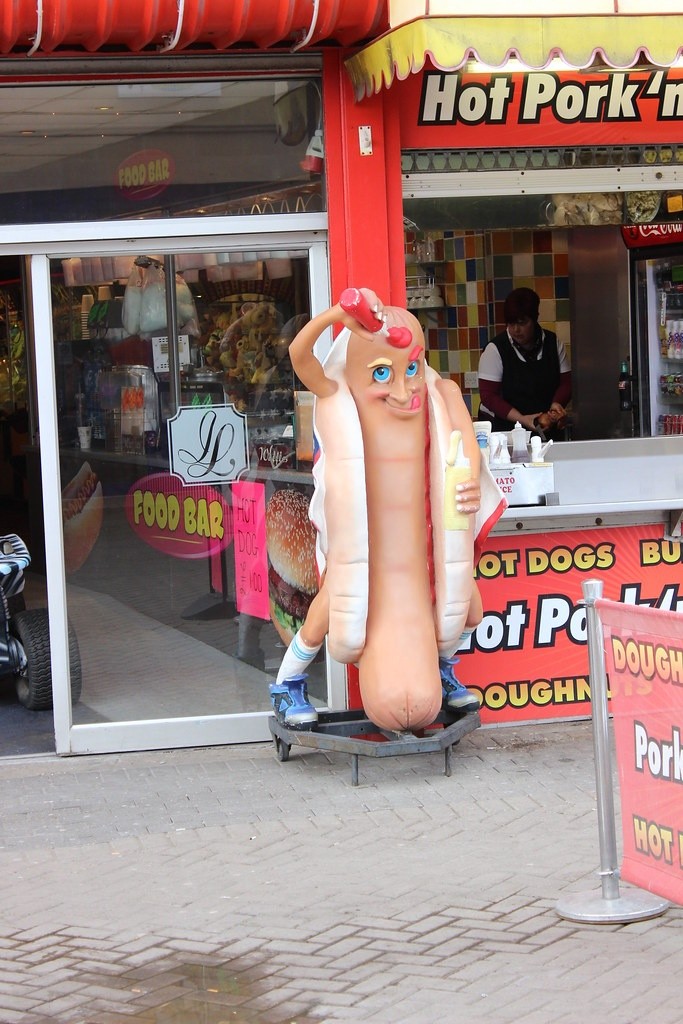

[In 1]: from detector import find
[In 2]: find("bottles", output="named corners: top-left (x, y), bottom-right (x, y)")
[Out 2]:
top-left (619, 361), bottom-right (632, 411)
top-left (339, 287), bottom-right (391, 339)
top-left (658, 414), bottom-right (683, 435)
top-left (512, 420), bottom-right (530, 463)
top-left (665, 319), bottom-right (683, 360)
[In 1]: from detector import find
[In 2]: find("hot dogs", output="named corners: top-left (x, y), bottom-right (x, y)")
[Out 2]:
top-left (265, 490), bottom-right (319, 649)
top-left (309, 307), bottom-right (479, 732)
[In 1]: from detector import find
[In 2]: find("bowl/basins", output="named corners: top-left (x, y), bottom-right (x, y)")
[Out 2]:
top-left (406, 285), bottom-right (443, 308)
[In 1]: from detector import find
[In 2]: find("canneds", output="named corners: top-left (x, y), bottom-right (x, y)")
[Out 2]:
top-left (660, 372), bottom-right (683, 400)
top-left (658, 414), bottom-right (683, 435)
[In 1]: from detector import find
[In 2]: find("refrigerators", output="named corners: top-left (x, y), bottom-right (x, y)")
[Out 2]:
top-left (623, 222), bottom-right (683, 438)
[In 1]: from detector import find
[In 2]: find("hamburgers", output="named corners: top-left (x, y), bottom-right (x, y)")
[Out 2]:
top-left (62, 461), bottom-right (104, 572)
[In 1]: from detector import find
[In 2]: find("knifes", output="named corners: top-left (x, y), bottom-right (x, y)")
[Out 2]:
top-left (532, 419), bottom-right (547, 440)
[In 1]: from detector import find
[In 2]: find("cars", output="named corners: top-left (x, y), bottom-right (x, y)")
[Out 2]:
top-left (86, 403), bottom-right (161, 453)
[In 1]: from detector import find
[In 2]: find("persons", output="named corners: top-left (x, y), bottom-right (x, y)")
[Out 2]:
top-left (478, 287), bottom-right (573, 441)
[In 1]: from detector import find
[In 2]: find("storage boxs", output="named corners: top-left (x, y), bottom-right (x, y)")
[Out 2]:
top-left (489, 462), bottom-right (555, 506)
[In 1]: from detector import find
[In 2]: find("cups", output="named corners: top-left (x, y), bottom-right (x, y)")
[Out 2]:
top-left (97, 287), bottom-right (112, 301)
top-left (81, 294), bottom-right (94, 340)
top-left (77, 426), bottom-right (91, 449)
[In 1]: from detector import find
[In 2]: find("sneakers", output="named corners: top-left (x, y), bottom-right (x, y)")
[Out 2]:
top-left (440, 658), bottom-right (480, 712)
top-left (269, 673), bottom-right (319, 729)
